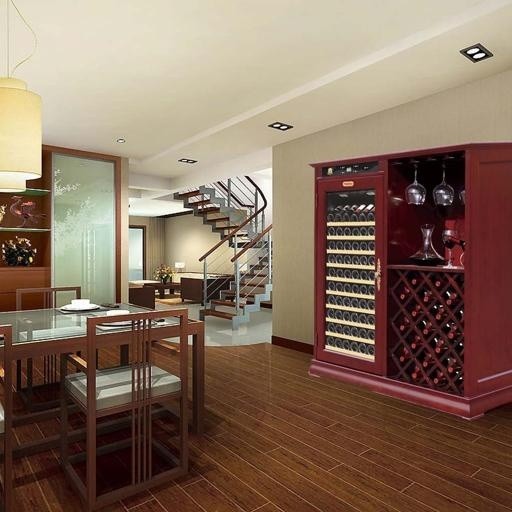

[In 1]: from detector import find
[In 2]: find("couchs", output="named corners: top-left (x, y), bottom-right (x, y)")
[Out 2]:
top-left (170, 272), bottom-right (230, 302)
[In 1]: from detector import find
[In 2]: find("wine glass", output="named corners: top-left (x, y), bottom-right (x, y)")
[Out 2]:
top-left (403, 165), bottom-right (467, 208)
top-left (441, 228), bottom-right (458, 267)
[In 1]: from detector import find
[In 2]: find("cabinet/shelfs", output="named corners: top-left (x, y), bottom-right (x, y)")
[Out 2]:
top-left (307, 141), bottom-right (512, 421)
top-left (1, 188), bottom-right (52, 267)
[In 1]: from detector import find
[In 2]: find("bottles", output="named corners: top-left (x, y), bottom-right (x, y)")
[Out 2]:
top-left (409, 224), bottom-right (447, 266)
top-left (322, 201), bottom-right (376, 360)
top-left (399, 276), bottom-right (463, 389)
top-left (447, 236), bottom-right (465, 252)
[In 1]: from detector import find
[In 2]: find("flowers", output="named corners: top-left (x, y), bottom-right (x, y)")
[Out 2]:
top-left (153, 264), bottom-right (176, 280)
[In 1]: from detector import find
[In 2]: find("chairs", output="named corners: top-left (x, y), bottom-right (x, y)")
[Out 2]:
top-left (16, 287), bottom-right (84, 407)
top-left (64, 308), bottom-right (194, 506)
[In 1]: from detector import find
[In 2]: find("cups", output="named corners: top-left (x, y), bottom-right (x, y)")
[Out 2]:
top-left (107, 310), bottom-right (128, 320)
top-left (72, 299), bottom-right (90, 309)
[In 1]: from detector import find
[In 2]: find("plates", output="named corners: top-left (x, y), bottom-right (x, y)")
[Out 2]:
top-left (99, 321), bottom-right (141, 326)
top-left (60, 304), bottom-right (98, 311)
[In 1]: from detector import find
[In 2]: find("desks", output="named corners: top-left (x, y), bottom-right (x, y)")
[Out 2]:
top-left (1, 302), bottom-right (205, 468)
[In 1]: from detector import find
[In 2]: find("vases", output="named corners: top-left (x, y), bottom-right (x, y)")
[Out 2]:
top-left (162, 278), bottom-right (168, 284)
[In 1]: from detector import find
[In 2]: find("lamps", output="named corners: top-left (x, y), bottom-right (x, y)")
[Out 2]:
top-left (2, 0), bottom-right (42, 195)
